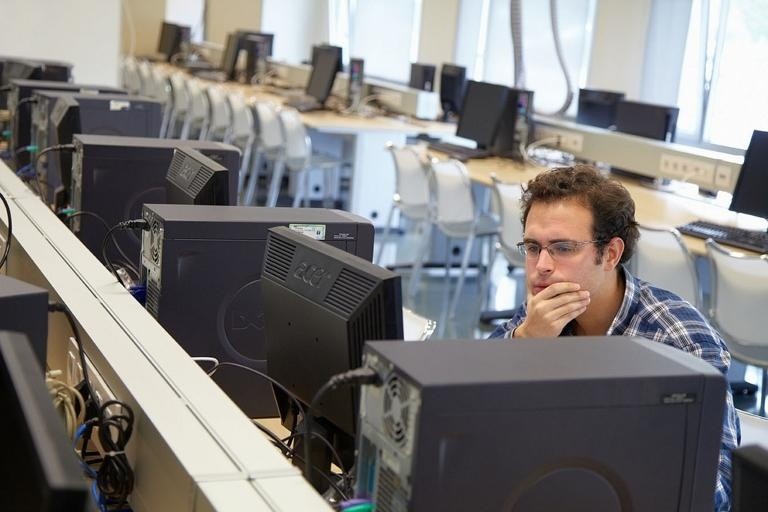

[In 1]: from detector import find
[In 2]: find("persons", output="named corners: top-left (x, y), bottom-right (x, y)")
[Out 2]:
top-left (480, 162), bottom-right (748, 510)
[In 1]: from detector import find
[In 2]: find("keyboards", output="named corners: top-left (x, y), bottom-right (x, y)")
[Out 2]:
top-left (677, 217), bottom-right (768, 255)
top-left (284, 92), bottom-right (324, 113)
top-left (406, 135), bottom-right (491, 164)
top-left (191, 68), bottom-right (229, 82)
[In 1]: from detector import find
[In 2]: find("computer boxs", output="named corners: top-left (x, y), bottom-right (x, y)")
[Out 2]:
top-left (497, 87), bottom-right (534, 158)
top-left (178, 27), bottom-right (192, 57)
top-left (136, 202), bottom-right (375, 419)
top-left (609, 100), bottom-right (680, 188)
top-left (0, 56), bottom-right (163, 202)
top-left (0, 272), bottom-right (48, 383)
top-left (354, 334), bottom-right (728, 512)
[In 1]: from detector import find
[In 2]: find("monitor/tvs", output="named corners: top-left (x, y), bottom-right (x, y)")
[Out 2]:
top-left (729, 129), bottom-right (768, 220)
top-left (258, 224), bottom-right (405, 497)
top-left (163, 143), bottom-right (230, 206)
top-left (50, 94), bottom-right (82, 225)
top-left (306, 44), bottom-right (343, 105)
top-left (575, 88), bottom-right (625, 169)
top-left (407, 61), bottom-right (510, 148)
top-left (0, 328), bottom-right (90, 512)
top-left (730, 445), bottom-right (768, 512)
top-left (68, 134), bottom-right (240, 284)
top-left (157, 22), bottom-right (182, 61)
top-left (239, 30), bottom-right (273, 80)
top-left (219, 36), bottom-right (241, 78)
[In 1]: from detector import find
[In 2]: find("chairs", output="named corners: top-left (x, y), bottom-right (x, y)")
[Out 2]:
top-left (67, 380), bottom-right (100, 423)
top-left (113, 49), bottom-right (767, 419)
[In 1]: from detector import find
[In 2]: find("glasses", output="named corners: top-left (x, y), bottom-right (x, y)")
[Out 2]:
top-left (516, 239), bottom-right (607, 262)
